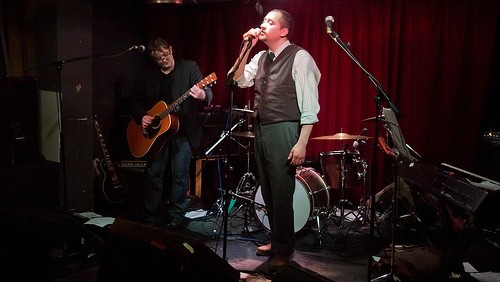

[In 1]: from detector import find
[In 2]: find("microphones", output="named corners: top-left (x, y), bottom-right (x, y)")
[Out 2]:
top-left (131, 45), bottom-right (146, 54)
top-left (353, 128), bottom-right (367, 148)
top-left (324, 16), bottom-right (334, 35)
top-left (244, 28), bottom-right (261, 42)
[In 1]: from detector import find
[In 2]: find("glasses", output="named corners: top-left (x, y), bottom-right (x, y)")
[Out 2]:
top-left (154, 48), bottom-right (171, 61)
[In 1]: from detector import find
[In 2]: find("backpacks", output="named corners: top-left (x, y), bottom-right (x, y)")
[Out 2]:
top-left (478, 223), bottom-right (500, 271)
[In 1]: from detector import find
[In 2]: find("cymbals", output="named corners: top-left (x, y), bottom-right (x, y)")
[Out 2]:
top-left (358, 115), bottom-right (385, 123)
top-left (311, 132), bottom-right (374, 140)
top-left (229, 130), bottom-right (255, 138)
top-left (232, 108), bottom-right (255, 115)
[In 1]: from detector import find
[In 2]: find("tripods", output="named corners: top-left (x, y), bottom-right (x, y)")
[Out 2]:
top-left (311, 139), bottom-right (431, 247)
top-left (228, 110), bottom-right (266, 248)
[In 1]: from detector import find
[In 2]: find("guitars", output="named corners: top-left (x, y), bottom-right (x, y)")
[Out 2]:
top-left (92, 114), bottom-right (127, 203)
top-left (127, 72), bottom-right (218, 159)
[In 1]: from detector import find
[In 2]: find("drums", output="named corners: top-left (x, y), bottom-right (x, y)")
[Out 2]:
top-left (319, 149), bottom-right (364, 190)
top-left (252, 166), bottom-right (331, 239)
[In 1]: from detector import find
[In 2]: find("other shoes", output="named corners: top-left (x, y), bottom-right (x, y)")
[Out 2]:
top-left (270, 249), bottom-right (295, 269)
top-left (169, 215), bottom-right (187, 229)
top-left (255, 242), bottom-right (271, 255)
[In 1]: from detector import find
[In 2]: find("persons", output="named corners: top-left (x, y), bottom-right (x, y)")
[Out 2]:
top-left (142, 37), bottom-right (212, 216)
top-left (227, 9), bottom-right (322, 270)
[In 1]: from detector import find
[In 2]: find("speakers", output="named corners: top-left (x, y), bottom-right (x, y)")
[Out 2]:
top-left (195, 159), bottom-right (226, 205)
top-left (117, 160), bottom-right (147, 187)
top-left (96, 219), bottom-right (241, 282)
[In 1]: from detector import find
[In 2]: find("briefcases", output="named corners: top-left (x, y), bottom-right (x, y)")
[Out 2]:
top-left (427, 161), bottom-right (500, 226)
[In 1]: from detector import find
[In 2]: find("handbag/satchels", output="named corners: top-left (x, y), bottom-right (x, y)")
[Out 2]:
top-left (370, 241), bottom-right (458, 282)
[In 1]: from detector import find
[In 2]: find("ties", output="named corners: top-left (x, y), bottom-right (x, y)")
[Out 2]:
top-left (268, 52), bottom-right (275, 64)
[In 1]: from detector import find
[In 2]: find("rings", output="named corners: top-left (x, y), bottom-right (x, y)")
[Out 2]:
top-left (300, 160), bottom-right (302, 163)
